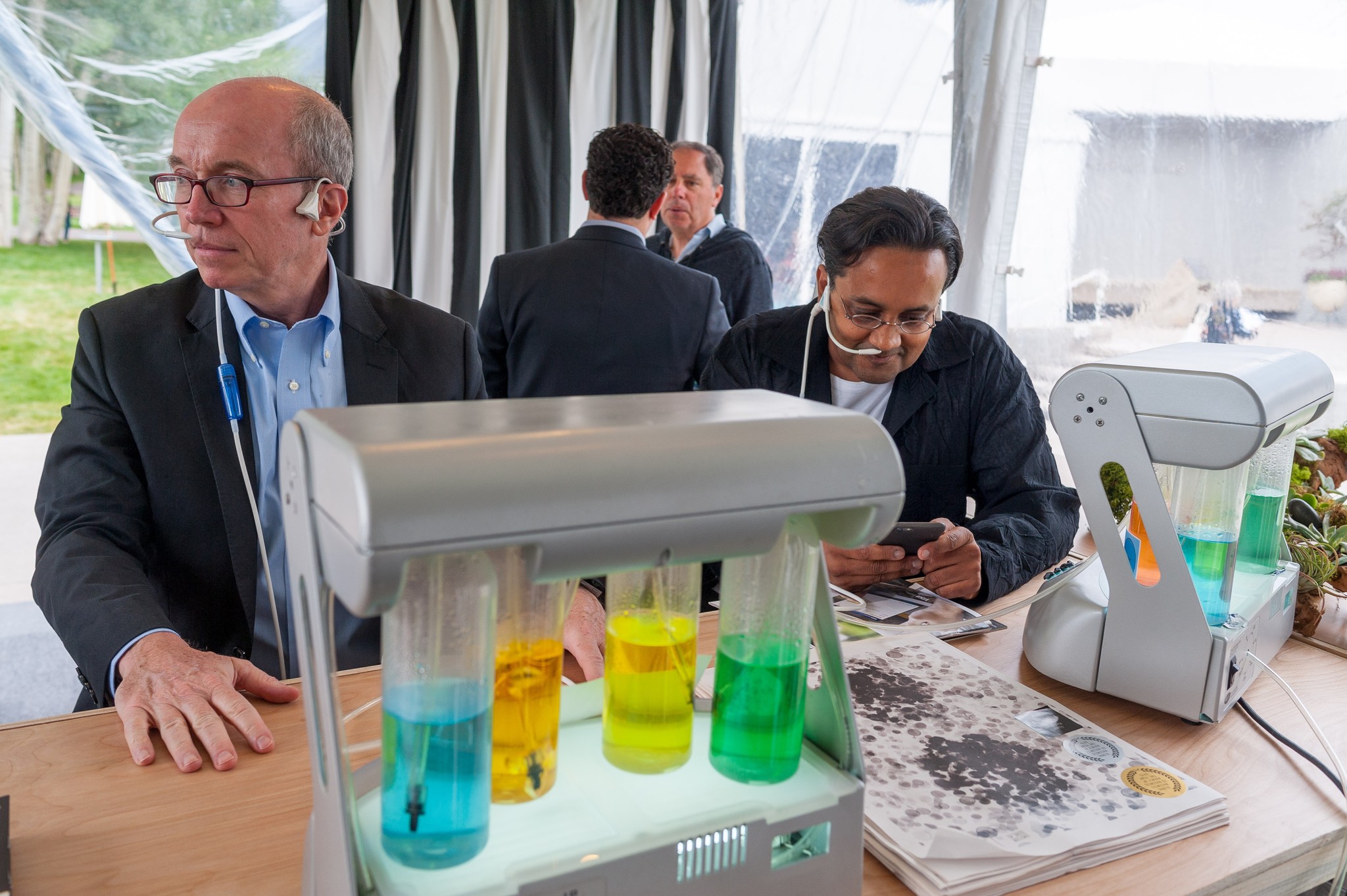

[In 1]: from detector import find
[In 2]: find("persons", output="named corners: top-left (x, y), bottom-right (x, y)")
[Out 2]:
top-left (638, 139), bottom-right (774, 329)
top-left (697, 205), bottom-right (1084, 618)
top-left (29, 77), bottom-right (490, 775)
top-left (475, 124), bottom-right (742, 398)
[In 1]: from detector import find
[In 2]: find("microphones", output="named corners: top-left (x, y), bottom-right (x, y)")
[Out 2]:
top-left (149, 210), bottom-right (193, 242)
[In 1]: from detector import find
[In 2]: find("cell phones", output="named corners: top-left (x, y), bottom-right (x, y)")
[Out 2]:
top-left (882, 520), bottom-right (944, 562)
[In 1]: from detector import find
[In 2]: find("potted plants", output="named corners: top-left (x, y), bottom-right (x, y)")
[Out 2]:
top-left (1283, 416), bottom-right (1346, 638)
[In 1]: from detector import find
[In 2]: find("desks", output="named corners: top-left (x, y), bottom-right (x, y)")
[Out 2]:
top-left (0, 519), bottom-right (1347, 896)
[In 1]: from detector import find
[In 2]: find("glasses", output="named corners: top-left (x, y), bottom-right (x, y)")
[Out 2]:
top-left (834, 284), bottom-right (943, 334)
top-left (148, 172), bottom-right (328, 208)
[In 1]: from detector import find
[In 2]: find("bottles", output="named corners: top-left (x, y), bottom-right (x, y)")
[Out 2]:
top-left (711, 533), bottom-right (819, 790)
top-left (494, 545), bottom-right (561, 807)
top-left (379, 548), bottom-right (497, 871)
top-left (1128, 433), bottom-right (1297, 625)
top-left (604, 565), bottom-right (701, 774)
